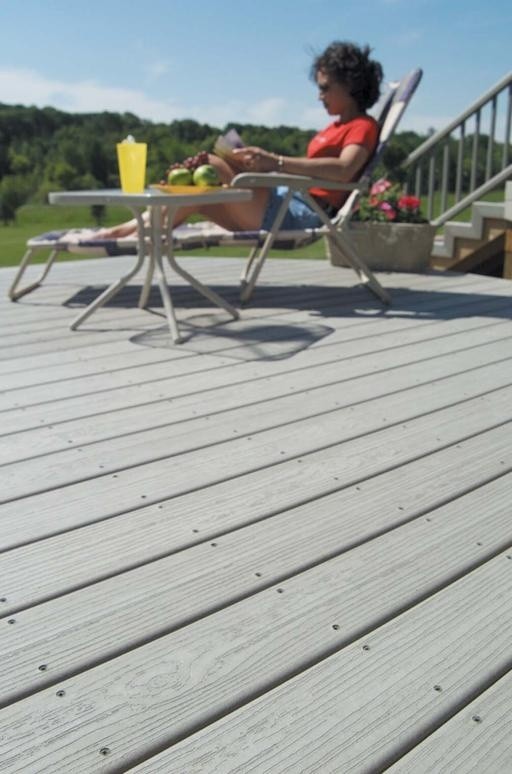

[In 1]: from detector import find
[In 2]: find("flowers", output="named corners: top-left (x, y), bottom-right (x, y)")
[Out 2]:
top-left (347, 178), bottom-right (430, 223)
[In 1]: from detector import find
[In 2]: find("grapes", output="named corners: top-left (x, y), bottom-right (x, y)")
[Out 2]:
top-left (160, 179), bottom-right (165, 186)
top-left (166, 151), bottom-right (209, 176)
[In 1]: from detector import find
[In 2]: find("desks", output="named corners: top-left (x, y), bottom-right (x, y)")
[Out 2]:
top-left (47, 188), bottom-right (254, 342)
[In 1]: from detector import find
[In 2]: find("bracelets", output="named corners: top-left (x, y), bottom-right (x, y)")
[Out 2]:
top-left (278, 155), bottom-right (284, 170)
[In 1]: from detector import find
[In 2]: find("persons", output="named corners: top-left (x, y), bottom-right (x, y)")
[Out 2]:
top-left (90, 41), bottom-right (383, 239)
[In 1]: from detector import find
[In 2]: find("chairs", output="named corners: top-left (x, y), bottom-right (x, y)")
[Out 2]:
top-left (7, 68), bottom-right (425, 305)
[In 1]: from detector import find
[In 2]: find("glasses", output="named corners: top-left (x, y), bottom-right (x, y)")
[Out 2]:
top-left (319, 84), bottom-right (329, 91)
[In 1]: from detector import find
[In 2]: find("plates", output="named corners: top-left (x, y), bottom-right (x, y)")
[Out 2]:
top-left (153, 184), bottom-right (222, 193)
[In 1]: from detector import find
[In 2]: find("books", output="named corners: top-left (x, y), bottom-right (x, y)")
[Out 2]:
top-left (214, 128), bottom-right (250, 170)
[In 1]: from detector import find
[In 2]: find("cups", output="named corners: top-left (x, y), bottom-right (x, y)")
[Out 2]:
top-left (116, 142), bottom-right (147, 194)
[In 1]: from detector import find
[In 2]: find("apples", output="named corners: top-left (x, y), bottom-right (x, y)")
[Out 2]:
top-left (194, 165), bottom-right (219, 186)
top-left (168, 168), bottom-right (191, 185)
